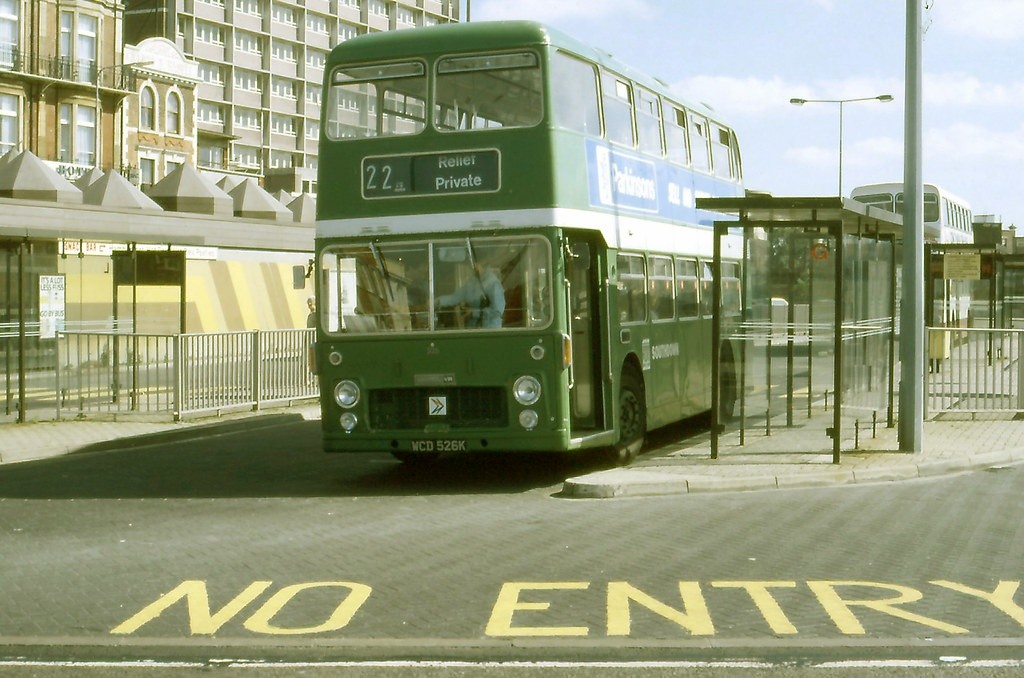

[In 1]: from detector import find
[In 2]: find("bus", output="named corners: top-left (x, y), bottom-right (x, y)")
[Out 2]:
top-left (848, 181), bottom-right (976, 342)
top-left (290, 21), bottom-right (748, 468)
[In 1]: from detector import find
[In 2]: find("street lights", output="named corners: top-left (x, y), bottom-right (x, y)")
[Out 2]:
top-left (790, 92), bottom-right (894, 199)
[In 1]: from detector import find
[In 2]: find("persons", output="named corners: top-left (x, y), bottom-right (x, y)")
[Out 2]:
top-left (425, 246), bottom-right (507, 329)
top-left (298, 287), bottom-right (358, 389)
top-left (624, 281), bottom-right (738, 322)
